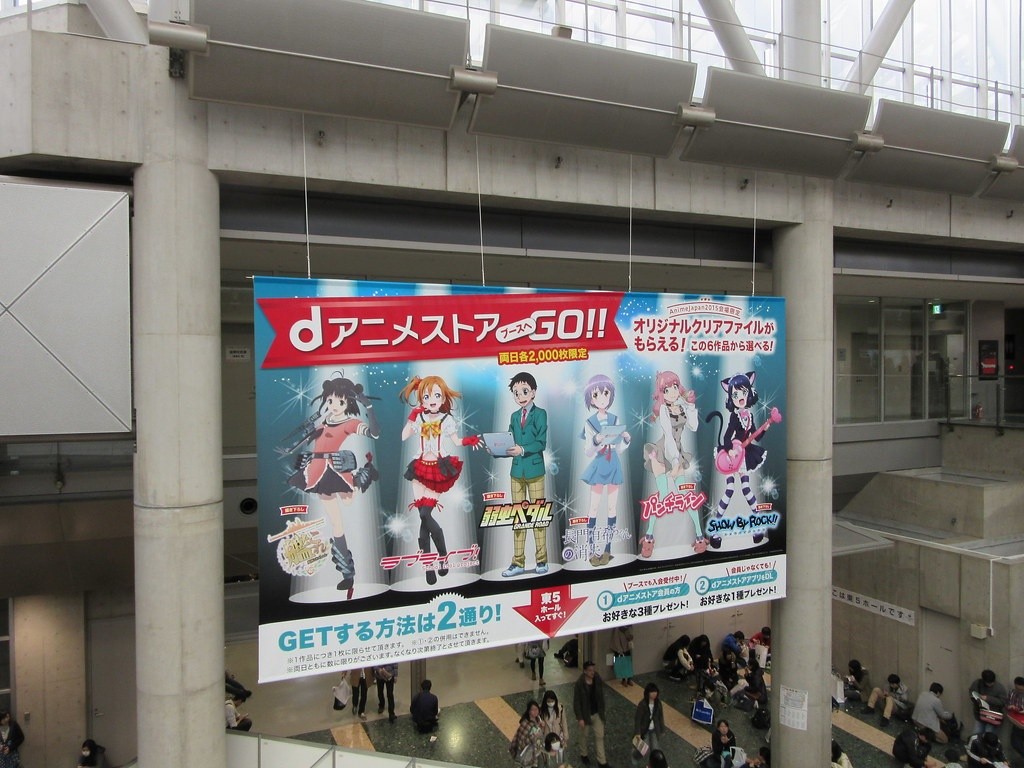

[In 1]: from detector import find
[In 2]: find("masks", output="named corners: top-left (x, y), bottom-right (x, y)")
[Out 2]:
top-left (546, 701), bottom-right (555, 707)
top-left (551, 741), bottom-right (561, 751)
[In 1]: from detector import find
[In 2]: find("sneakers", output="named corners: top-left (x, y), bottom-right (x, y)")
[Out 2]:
top-left (631, 751), bottom-right (639, 765)
top-left (670, 674), bottom-right (682, 680)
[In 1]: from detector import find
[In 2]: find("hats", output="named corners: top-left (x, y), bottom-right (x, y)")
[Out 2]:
top-left (583, 660), bottom-right (595, 667)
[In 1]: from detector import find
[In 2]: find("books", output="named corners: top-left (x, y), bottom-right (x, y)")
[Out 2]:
top-left (971, 691), bottom-right (989, 710)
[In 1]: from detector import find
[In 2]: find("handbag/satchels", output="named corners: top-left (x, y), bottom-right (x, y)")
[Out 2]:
top-left (333, 677), bottom-right (353, 711)
top-left (614, 653), bottom-right (634, 678)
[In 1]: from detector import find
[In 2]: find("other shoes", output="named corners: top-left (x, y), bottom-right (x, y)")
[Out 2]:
top-left (521, 662), bottom-right (524, 668)
top-left (860, 706), bottom-right (874, 714)
top-left (352, 706), bottom-right (357, 715)
top-left (515, 658), bottom-right (519, 663)
top-left (622, 680), bottom-right (628, 687)
top-left (532, 673), bottom-right (537, 680)
top-left (539, 678), bottom-right (546, 685)
top-left (378, 707), bottom-right (384, 713)
top-left (582, 755), bottom-right (589, 765)
top-left (360, 713), bottom-right (367, 718)
top-left (598, 762), bottom-right (609, 768)
top-left (389, 712), bottom-right (397, 721)
top-left (627, 680), bottom-right (634, 686)
top-left (880, 717), bottom-right (888, 727)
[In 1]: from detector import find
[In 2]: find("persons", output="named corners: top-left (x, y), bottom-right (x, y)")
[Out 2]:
top-left (611, 625), bottom-right (633, 686)
top-left (574, 661), bottom-right (611, 768)
top-left (225, 671), bottom-right (252, 731)
top-left (515, 639), bottom-right (550, 685)
top-left (832, 740), bottom-right (854, 768)
top-left (77, 739), bottom-right (107, 768)
top-left (893, 727), bottom-right (935, 768)
top-left (517, 689), bottom-right (573, 768)
top-left (631, 683), bottom-right (665, 765)
top-left (412, 680), bottom-right (438, 731)
top-left (558, 634), bottom-right (578, 667)
top-left (650, 750), bottom-right (668, 768)
top-left (712, 720), bottom-right (771, 768)
top-left (911, 683), bottom-right (954, 744)
top-left (341, 663), bottom-right (398, 720)
top-left (860, 674), bottom-right (907, 727)
top-left (664, 627), bottom-right (771, 708)
top-left (0, 709), bottom-right (25, 768)
top-left (844, 660), bottom-right (869, 702)
top-left (945, 671), bottom-right (1024, 768)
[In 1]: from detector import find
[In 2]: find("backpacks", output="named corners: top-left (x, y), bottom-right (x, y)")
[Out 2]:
top-left (752, 708), bottom-right (770, 729)
